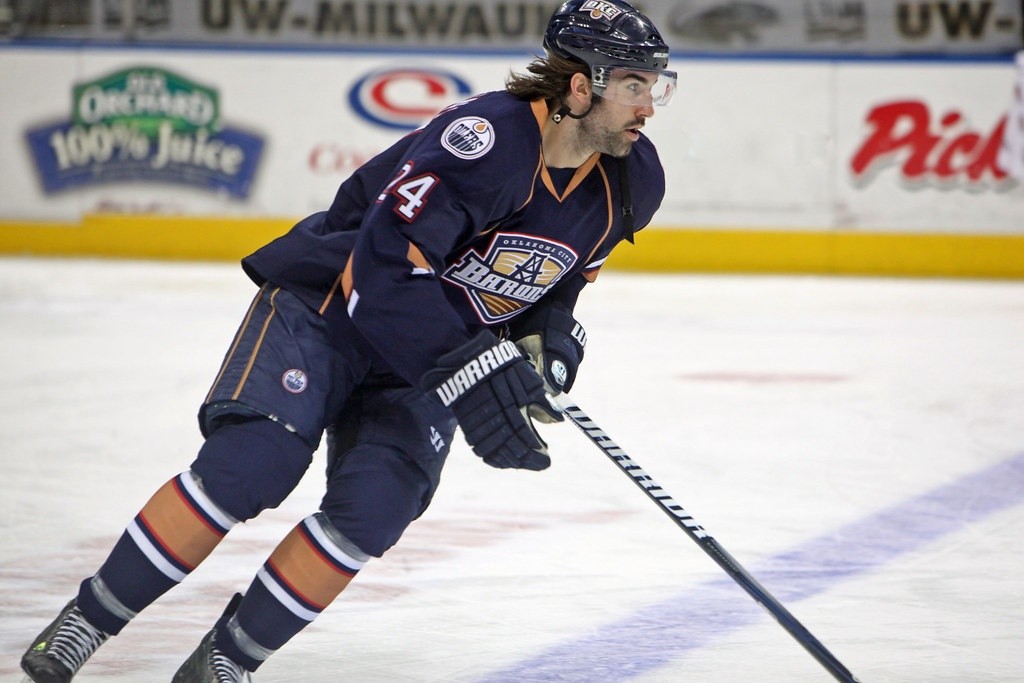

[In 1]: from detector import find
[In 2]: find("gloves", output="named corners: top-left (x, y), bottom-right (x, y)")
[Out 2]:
top-left (504, 300), bottom-right (587, 397)
top-left (419, 330), bottom-right (565, 471)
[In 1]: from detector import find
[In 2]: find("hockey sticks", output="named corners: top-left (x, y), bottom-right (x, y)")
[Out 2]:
top-left (528, 355), bottom-right (861, 683)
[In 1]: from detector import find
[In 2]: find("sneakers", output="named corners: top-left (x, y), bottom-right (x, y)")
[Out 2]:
top-left (19, 596), bottom-right (131, 683)
top-left (171, 592), bottom-right (252, 683)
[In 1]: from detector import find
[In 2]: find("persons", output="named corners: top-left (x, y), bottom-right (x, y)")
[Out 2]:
top-left (17, 1), bottom-right (677, 683)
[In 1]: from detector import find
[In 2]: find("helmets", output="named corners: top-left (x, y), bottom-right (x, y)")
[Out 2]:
top-left (541, 0), bottom-right (669, 106)
